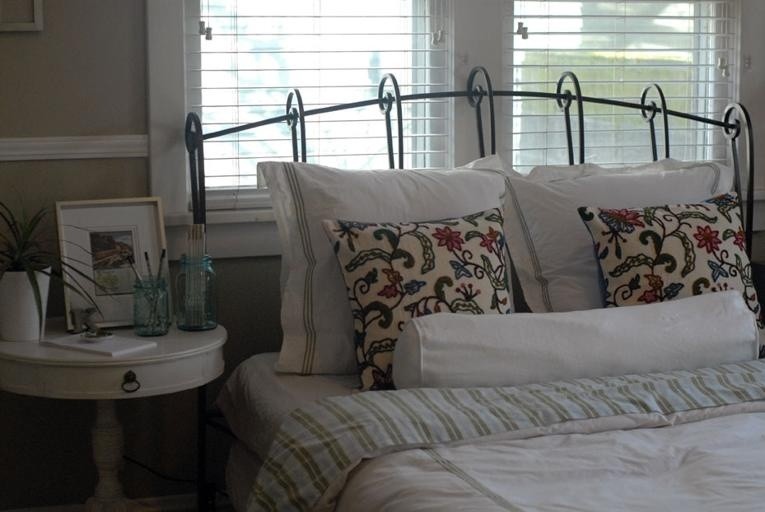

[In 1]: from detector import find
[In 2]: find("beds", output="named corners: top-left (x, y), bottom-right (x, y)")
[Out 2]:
top-left (179, 69), bottom-right (763, 512)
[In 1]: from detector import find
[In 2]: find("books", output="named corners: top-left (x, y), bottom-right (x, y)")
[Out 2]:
top-left (40, 329), bottom-right (158, 358)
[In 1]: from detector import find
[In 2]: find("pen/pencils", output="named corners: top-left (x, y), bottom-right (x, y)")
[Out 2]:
top-left (127, 249), bottom-right (166, 331)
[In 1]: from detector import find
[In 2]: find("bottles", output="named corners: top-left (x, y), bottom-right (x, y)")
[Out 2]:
top-left (133, 276), bottom-right (166, 337)
top-left (176, 254), bottom-right (218, 331)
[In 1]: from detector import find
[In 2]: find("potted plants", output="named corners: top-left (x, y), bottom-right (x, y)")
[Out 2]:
top-left (0, 191), bottom-right (104, 344)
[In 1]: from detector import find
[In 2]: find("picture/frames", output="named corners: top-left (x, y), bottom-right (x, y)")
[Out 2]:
top-left (0, 0), bottom-right (44, 33)
top-left (55, 197), bottom-right (172, 332)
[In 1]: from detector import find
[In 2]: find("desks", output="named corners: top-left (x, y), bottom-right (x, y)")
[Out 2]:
top-left (0, 318), bottom-right (229, 512)
